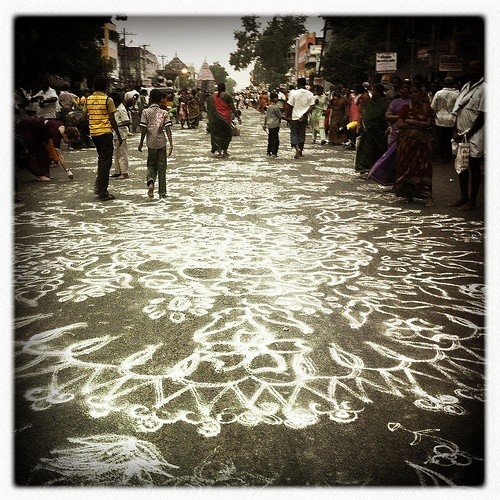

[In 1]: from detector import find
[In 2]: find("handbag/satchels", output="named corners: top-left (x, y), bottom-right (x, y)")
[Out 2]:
top-left (454, 134), bottom-right (469, 175)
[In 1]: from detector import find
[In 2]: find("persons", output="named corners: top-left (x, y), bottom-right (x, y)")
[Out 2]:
top-left (14, 71), bottom-right (469, 198)
top-left (80, 75), bottom-right (123, 201)
top-left (447, 60), bottom-right (485, 210)
top-left (394, 82), bottom-right (438, 207)
top-left (138, 89), bottom-right (173, 199)
top-left (286, 78), bottom-right (315, 159)
top-left (108, 92), bottom-right (131, 180)
top-left (207, 82), bottom-right (242, 156)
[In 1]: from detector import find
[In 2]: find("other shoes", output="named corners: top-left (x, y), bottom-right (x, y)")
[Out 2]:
top-left (36, 176), bottom-right (52, 181)
top-left (109, 173), bottom-right (121, 177)
top-left (343, 143), bottom-right (356, 151)
top-left (119, 174), bottom-right (129, 179)
top-left (101, 194), bottom-right (115, 201)
top-left (65, 168), bottom-right (73, 179)
